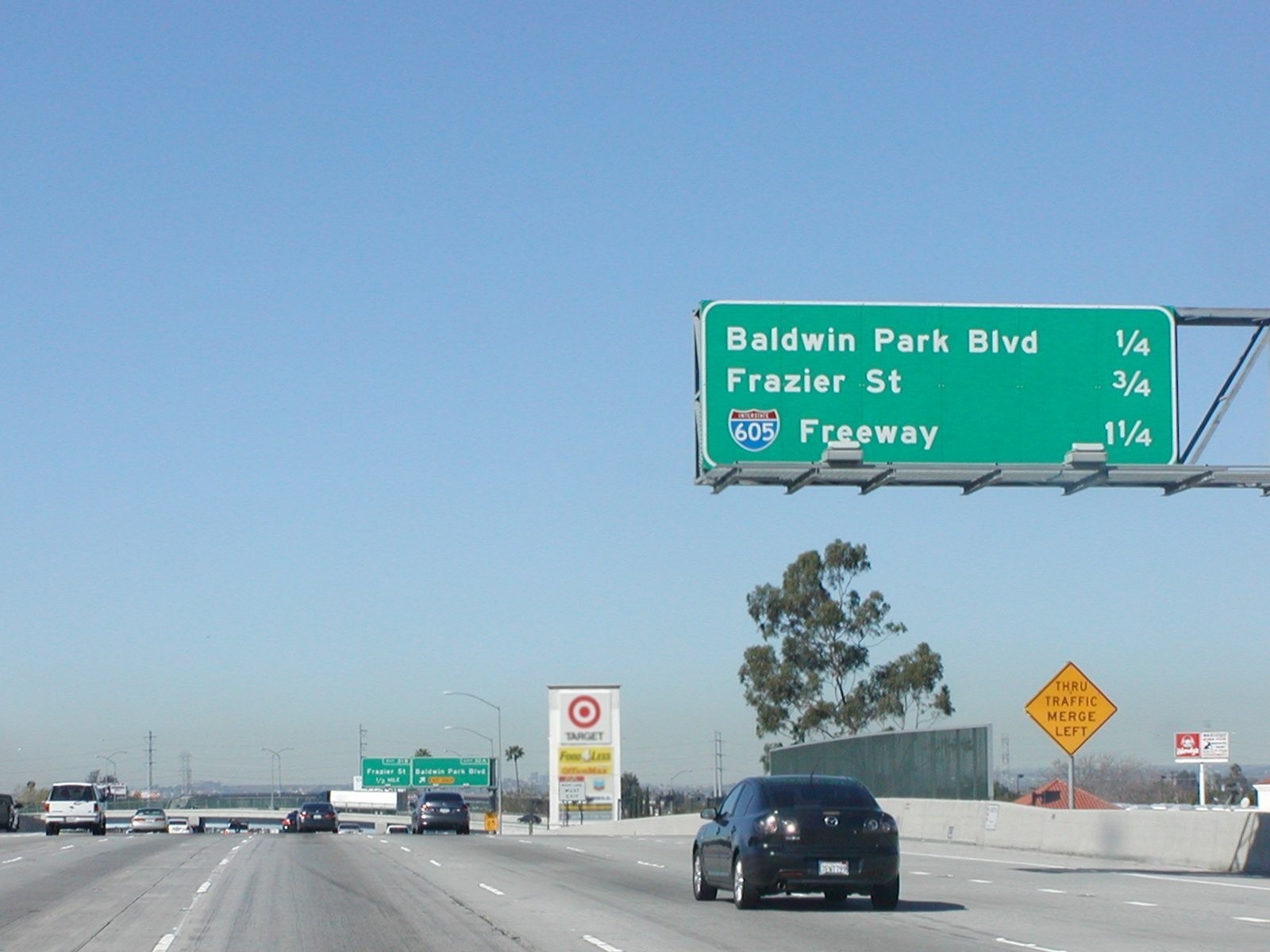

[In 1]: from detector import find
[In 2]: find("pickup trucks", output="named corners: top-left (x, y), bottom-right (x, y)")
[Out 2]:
top-left (41, 782), bottom-right (107, 836)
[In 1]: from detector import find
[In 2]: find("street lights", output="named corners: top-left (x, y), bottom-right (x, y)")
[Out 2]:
top-left (260, 747), bottom-right (281, 811)
top-left (104, 751), bottom-right (128, 807)
top-left (269, 747), bottom-right (295, 811)
top-left (96, 755), bottom-right (117, 805)
top-left (440, 689), bottom-right (502, 836)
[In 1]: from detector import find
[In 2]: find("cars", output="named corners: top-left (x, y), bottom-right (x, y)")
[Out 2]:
top-left (130, 808), bottom-right (168, 832)
top-left (167, 819), bottom-right (194, 834)
top-left (516, 814), bottom-right (543, 824)
top-left (384, 824), bottom-right (410, 834)
top-left (280, 802), bottom-right (340, 835)
top-left (337, 822), bottom-right (365, 836)
top-left (691, 775), bottom-right (901, 911)
top-left (407, 792), bottom-right (470, 835)
top-left (0, 794), bottom-right (23, 832)
top-left (224, 822), bottom-right (248, 834)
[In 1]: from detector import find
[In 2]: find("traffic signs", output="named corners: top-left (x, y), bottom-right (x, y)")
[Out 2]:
top-left (697, 299), bottom-right (1179, 480)
top-left (410, 755), bottom-right (491, 788)
top-left (360, 757), bottom-right (411, 790)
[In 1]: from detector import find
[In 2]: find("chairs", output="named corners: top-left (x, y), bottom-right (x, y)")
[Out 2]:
top-left (57, 789), bottom-right (69, 800)
top-left (71, 788), bottom-right (80, 801)
top-left (82, 788), bottom-right (92, 802)
